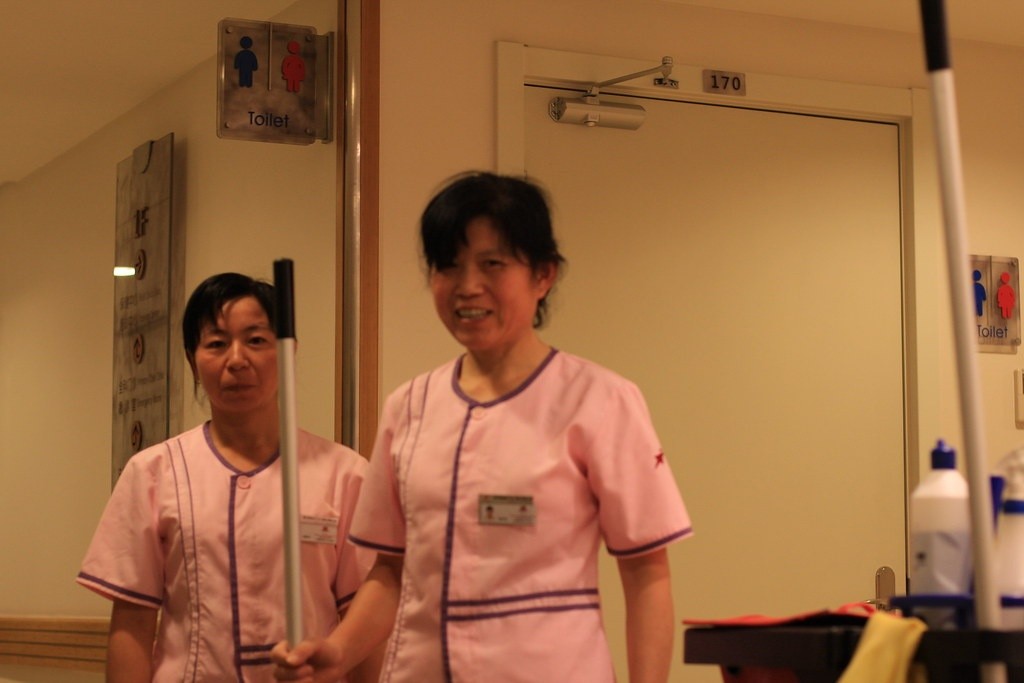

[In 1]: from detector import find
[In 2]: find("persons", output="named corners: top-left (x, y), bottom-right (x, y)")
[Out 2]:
top-left (269, 171), bottom-right (692, 682)
top-left (76, 272), bottom-right (390, 683)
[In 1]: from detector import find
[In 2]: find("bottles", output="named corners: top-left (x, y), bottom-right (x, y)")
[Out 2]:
top-left (905, 440), bottom-right (976, 601)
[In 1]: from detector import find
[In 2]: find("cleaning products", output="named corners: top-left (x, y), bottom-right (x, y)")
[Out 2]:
top-left (908, 438), bottom-right (972, 632)
top-left (989, 445), bottom-right (1024, 630)
